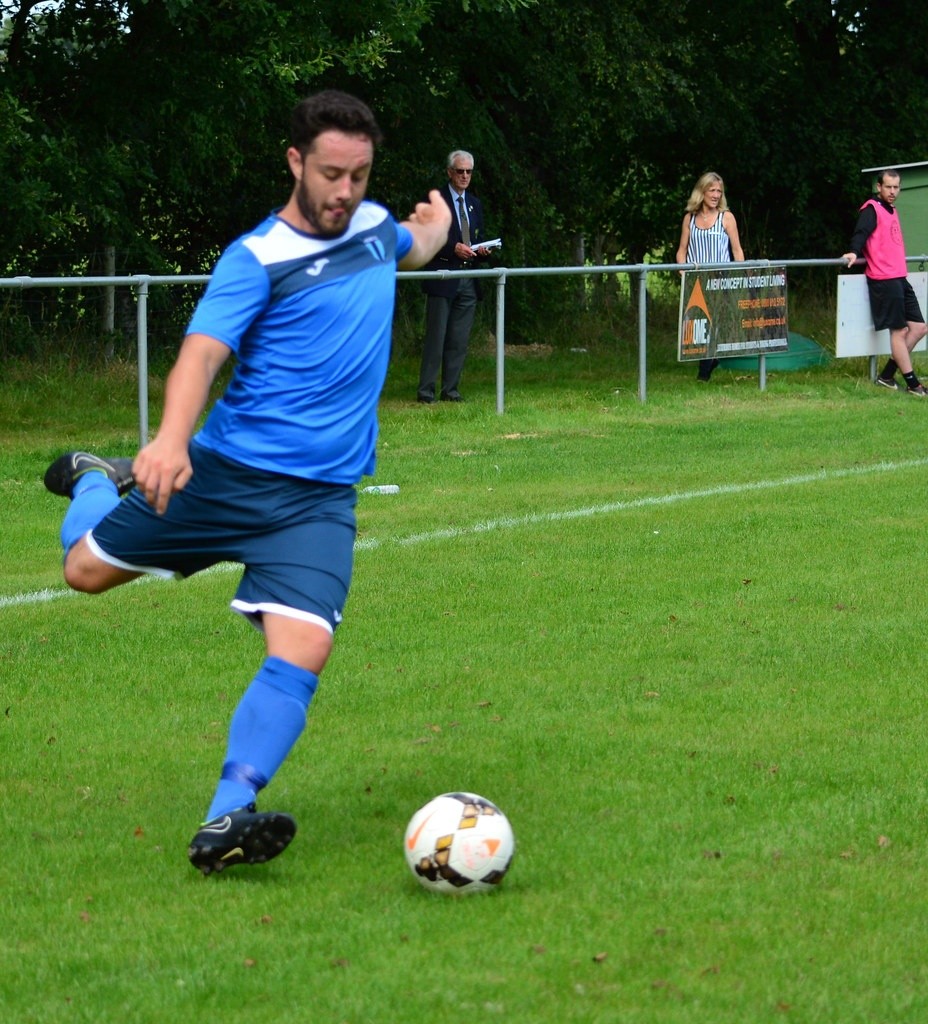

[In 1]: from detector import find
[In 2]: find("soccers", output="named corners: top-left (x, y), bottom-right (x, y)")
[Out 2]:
top-left (403, 790), bottom-right (516, 896)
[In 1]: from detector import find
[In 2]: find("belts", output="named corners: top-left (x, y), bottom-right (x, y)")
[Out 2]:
top-left (458, 261), bottom-right (473, 270)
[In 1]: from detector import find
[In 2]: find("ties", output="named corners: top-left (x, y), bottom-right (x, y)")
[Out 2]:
top-left (456, 197), bottom-right (471, 247)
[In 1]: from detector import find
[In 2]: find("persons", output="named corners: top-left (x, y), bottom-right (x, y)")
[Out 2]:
top-left (43, 91), bottom-right (453, 876)
top-left (840, 170), bottom-right (928, 397)
top-left (416, 150), bottom-right (491, 404)
top-left (677, 173), bottom-right (745, 381)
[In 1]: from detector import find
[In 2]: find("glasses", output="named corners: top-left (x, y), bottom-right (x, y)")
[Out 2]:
top-left (454, 169), bottom-right (473, 174)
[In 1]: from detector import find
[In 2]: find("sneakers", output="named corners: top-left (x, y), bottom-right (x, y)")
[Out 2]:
top-left (188, 801), bottom-right (297, 876)
top-left (43, 451), bottom-right (135, 499)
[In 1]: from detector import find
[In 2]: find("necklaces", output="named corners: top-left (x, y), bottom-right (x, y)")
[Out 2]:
top-left (703, 216), bottom-right (707, 220)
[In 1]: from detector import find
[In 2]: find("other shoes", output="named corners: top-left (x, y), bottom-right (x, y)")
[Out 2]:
top-left (417, 397), bottom-right (434, 404)
top-left (443, 396), bottom-right (464, 402)
top-left (877, 375), bottom-right (900, 390)
top-left (907, 383), bottom-right (928, 398)
